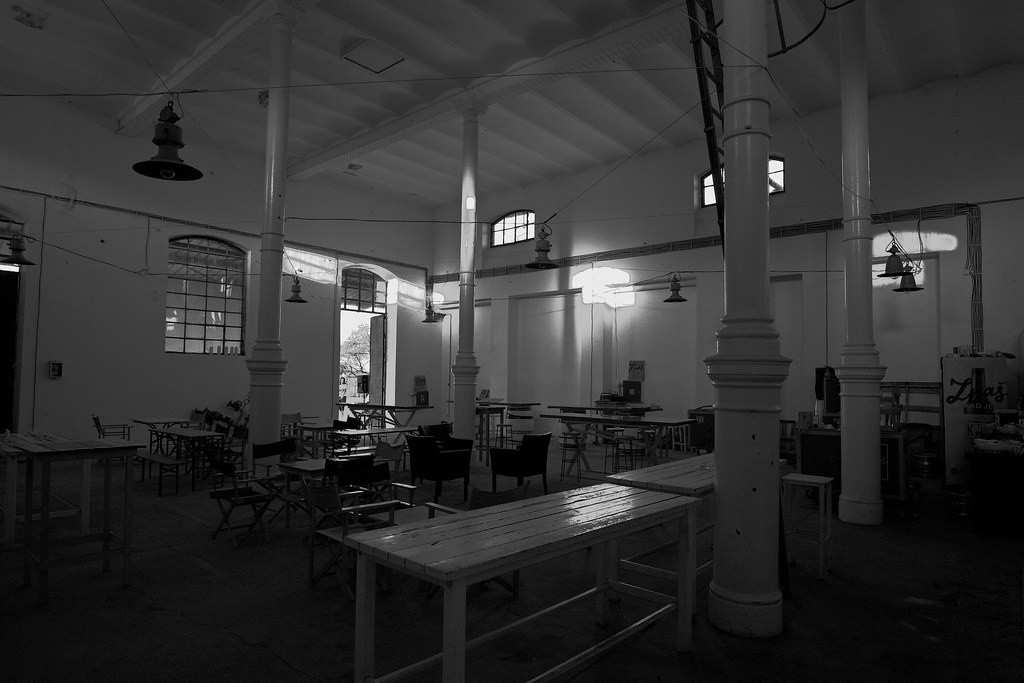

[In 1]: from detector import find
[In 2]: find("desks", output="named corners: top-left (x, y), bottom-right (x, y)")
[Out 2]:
top-left (559, 412), bottom-right (697, 469)
top-left (344, 455), bottom-right (787, 683)
top-left (0, 404), bottom-right (434, 604)
top-left (547, 406), bottom-right (662, 415)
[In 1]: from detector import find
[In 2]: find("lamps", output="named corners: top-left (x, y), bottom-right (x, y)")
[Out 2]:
top-left (662, 271), bottom-right (688, 303)
top-left (131, 100), bottom-right (204, 181)
top-left (877, 240), bottom-right (925, 294)
top-left (421, 302), bottom-right (439, 324)
top-left (526, 224), bottom-right (557, 269)
top-left (284, 276), bottom-right (308, 304)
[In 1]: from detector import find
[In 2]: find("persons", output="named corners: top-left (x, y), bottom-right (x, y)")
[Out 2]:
top-left (339, 377), bottom-right (348, 411)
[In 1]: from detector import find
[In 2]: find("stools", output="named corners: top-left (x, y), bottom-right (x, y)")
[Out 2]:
top-left (781, 473), bottom-right (835, 579)
top-left (559, 431), bottom-right (587, 482)
top-left (672, 424), bottom-right (697, 453)
top-left (605, 427), bottom-right (655, 474)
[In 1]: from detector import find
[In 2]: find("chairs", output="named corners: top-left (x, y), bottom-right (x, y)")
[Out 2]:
top-left (91, 407), bottom-right (553, 607)
